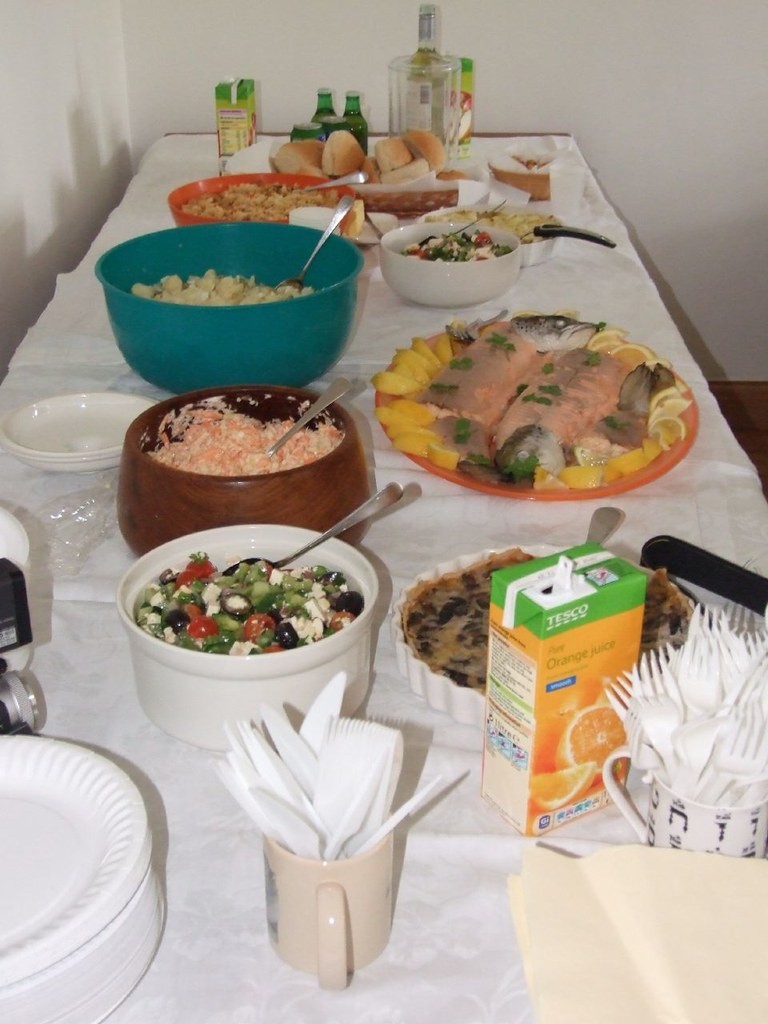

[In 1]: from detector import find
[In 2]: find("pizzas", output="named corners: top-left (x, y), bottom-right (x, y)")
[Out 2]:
top-left (400, 551), bottom-right (693, 692)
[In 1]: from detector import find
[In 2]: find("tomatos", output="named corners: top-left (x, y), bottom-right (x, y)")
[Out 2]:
top-left (242, 613), bottom-right (276, 641)
top-left (187, 615), bottom-right (219, 636)
top-left (175, 570), bottom-right (197, 588)
top-left (330, 612), bottom-right (356, 631)
top-left (185, 604), bottom-right (200, 618)
top-left (263, 646), bottom-right (284, 653)
top-left (185, 552), bottom-right (214, 577)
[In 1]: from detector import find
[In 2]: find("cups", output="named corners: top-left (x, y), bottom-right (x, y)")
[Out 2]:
top-left (262, 831), bottom-right (394, 992)
top-left (387, 53), bottom-right (461, 172)
top-left (289, 207), bottom-right (340, 238)
top-left (601, 746), bottom-right (767, 860)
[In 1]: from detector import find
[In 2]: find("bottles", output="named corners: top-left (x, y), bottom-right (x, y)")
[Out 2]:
top-left (343, 90), bottom-right (367, 155)
top-left (408, 4), bottom-right (445, 148)
top-left (311, 85), bottom-right (336, 123)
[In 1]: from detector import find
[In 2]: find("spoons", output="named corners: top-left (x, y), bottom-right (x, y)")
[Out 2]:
top-left (273, 193), bottom-right (356, 292)
top-left (205, 671), bottom-right (443, 858)
top-left (221, 481), bottom-right (404, 577)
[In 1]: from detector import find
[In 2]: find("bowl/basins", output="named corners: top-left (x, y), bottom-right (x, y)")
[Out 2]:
top-left (116, 523), bottom-right (379, 751)
top-left (94, 221), bottom-right (364, 393)
top-left (378, 221), bottom-right (520, 307)
top-left (167, 171), bottom-right (355, 236)
top-left (487, 155), bottom-right (551, 201)
top-left (118, 385), bottom-right (366, 551)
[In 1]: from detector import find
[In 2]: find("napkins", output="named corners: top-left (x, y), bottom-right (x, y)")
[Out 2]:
top-left (510, 843), bottom-right (768, 1024)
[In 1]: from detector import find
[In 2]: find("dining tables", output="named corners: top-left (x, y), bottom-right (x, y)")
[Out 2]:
top-left (0, 132), bottom-right (768, 1024)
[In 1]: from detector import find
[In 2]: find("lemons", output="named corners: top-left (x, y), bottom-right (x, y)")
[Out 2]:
top-left (373, 310), bottom-right (690, 492)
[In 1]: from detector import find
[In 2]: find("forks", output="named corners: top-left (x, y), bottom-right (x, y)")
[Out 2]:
top-left (604, 602), bottom-right (767, 806)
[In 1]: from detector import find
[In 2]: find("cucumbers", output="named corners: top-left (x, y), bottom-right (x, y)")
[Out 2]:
top-left (138, 560), bottom-right (342, 656)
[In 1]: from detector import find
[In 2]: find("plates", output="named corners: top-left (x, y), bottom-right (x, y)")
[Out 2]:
top-left (1, 730), bottom-right (165, 1024)
top-left (420, 202), bottom-right (560, 267)
top-left (1, 390), bottom-right (160, 475)
top-left (0, 507), bottom-right (29, 567)
top-left (376, 316), bottom-right (699, 500)
top-left (390, 543), bottom-right (698, 724)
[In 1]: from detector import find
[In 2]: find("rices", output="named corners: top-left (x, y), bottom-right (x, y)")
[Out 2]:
top-left (181, 182), bottom-right (343, 222)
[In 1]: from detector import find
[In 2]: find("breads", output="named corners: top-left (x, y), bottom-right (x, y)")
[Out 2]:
top-left (273, 128), bottom-right (467, 185)
